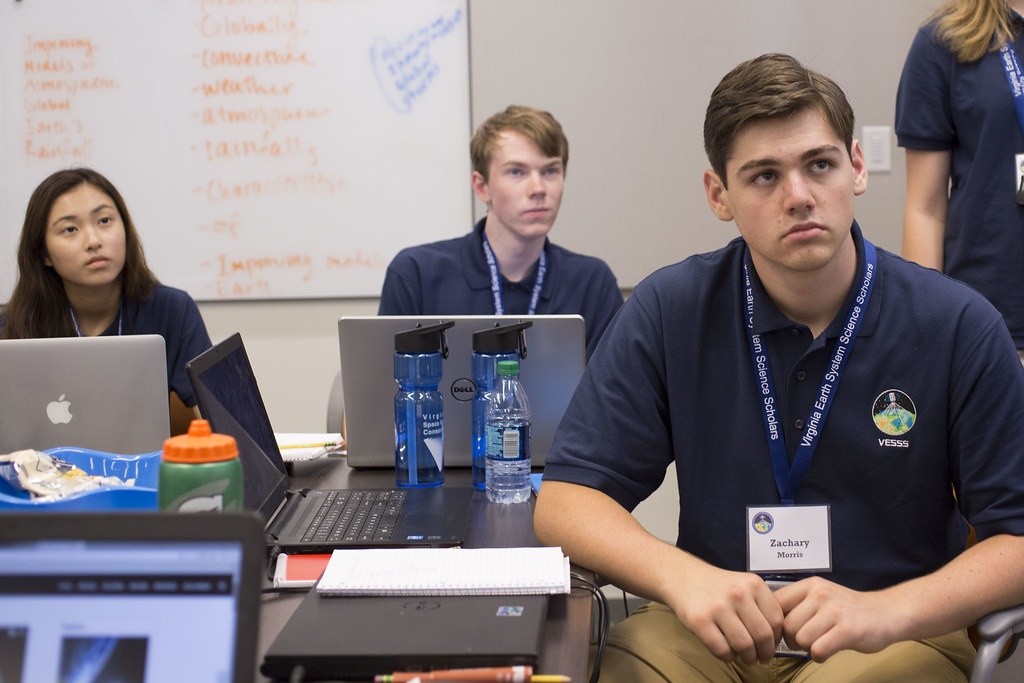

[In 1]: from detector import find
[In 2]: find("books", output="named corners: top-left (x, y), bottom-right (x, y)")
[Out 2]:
top-left (316, 546), bottom-right (572, 596)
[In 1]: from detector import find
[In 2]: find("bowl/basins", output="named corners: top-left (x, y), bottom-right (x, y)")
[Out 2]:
top-left (0, 447), bottom-right (168, 513)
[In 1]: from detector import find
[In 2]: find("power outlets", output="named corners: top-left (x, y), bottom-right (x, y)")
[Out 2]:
top-left (863, 123), bottom-right (891, 173)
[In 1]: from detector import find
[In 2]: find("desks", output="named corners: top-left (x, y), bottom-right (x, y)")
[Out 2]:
top-left (255, 456), bottom-right (593, 683)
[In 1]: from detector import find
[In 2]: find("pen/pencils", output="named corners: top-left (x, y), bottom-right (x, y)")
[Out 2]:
top-left (280, 442), bottom-right (337, 452)
top-left (374, 669), bottom-right (575, 683)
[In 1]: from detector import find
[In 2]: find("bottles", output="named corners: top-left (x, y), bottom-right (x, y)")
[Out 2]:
top-left (157, 419), bottom-right (244, 515)
top-left (484, 360), bottom-right (532, 504)
top-left (393, 318), bottom-right (456, 489)
top-left (471, 321), bottom-right (533, 490)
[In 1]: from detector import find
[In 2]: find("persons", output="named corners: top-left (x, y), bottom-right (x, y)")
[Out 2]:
top-left (896, 0), bottom-right (1024, 364)
top-left (535, 55), bottom-right (1024, 683)
top-left (1, 167), bottom-right (212, 436)
top-left (340, 104), bottom-right (625, 440)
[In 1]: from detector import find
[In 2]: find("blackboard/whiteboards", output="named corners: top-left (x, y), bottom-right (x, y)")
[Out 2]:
top-left (0, 0), bottom-right (475, 299)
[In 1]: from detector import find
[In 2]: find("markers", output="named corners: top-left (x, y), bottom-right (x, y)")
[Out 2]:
top-left (393, 666), bottom-right (533, 683)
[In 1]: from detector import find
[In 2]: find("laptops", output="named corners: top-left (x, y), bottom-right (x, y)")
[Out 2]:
top-left (338, 315), bottom-right (588, 468)
top-left (0, 505), bottom-right (263, 683)
top-left (0, 334), bottom-right (170, 455)
top-left (260, 568), bottom-right (549, 683)
top-left (185, 332), bottom-right (474, 550)
